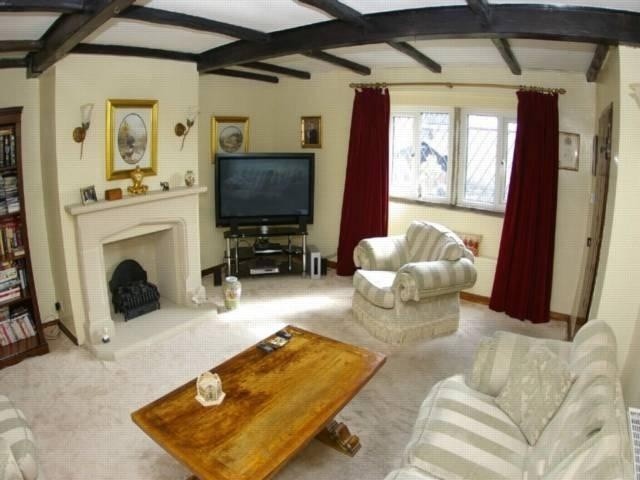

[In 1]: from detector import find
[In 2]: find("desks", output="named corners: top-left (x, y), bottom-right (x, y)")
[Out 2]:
top-left (224, 227), bottom-right (307, 278)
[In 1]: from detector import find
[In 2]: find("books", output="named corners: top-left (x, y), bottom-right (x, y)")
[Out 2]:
top-left (0, 127), bottom-right (41, 363)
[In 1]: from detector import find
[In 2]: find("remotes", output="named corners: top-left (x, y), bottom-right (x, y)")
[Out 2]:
top-left (256, 341), bottom-right (274, 353)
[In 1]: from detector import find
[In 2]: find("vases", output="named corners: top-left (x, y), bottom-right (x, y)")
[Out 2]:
top-left (222, 276), bottom-right (241, 308)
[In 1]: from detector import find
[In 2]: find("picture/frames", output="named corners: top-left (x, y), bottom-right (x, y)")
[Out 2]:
top-left (558, 130), bottom-right (580, 171)
top-left (210, 116), bottom-right (250, 164)
top-left (300, 116), bottom-right (322, 149)
top-left (105, 98), bottom-right (158, 181)
top-left (80, 184), bottom-right (98, 204)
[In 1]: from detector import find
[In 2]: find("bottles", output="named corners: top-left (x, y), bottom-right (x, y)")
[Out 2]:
top-left (184, 170), bottom-right (195, 187)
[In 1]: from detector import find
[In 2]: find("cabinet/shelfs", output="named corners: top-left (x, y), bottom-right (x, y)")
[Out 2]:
top-left (0, 105), bottom-right (50, 371)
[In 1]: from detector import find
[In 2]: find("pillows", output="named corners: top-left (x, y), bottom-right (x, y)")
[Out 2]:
top-left (493, 342), bottom-right (578, 447)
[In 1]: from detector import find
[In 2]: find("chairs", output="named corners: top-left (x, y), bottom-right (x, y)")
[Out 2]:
top-left (352, 220), bottom-right (478, 344)
top-left (0, 392), bottom-right (39, 480)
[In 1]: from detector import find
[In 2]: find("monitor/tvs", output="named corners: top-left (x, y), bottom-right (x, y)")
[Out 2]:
top-left (215, 152), bottom-right (314, 233)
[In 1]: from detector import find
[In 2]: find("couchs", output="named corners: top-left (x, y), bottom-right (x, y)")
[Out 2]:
top-left (387, 317), bottom-right (634, 480)
